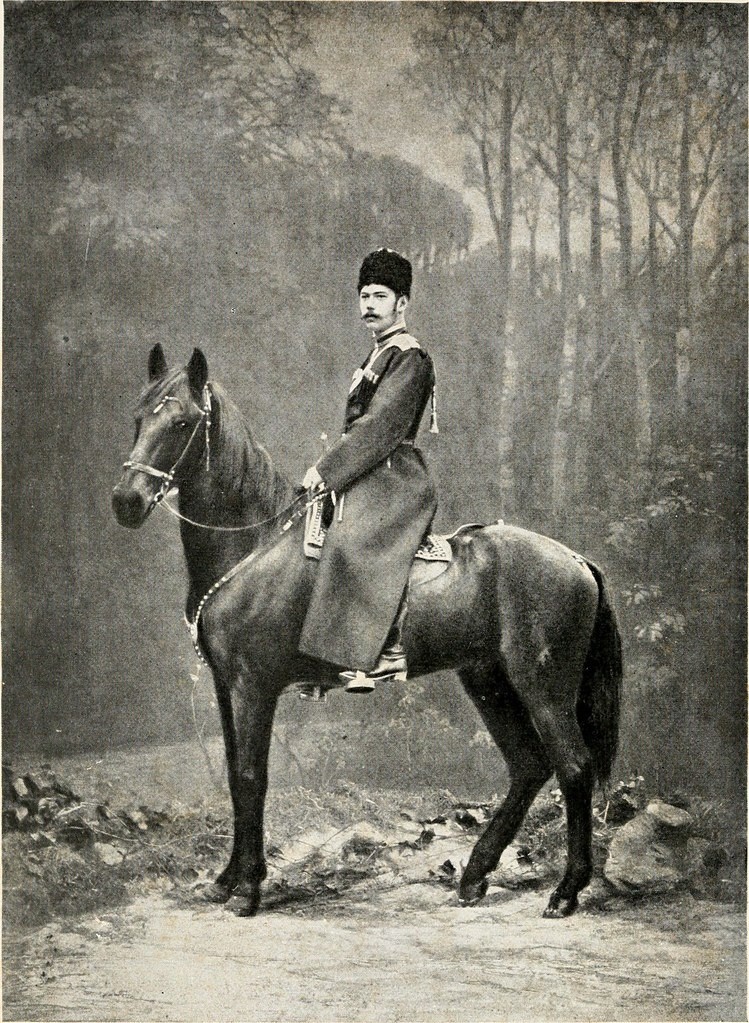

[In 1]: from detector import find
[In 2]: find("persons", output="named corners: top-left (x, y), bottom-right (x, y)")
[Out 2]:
top-left (297, 246), bottom-right (437, 705)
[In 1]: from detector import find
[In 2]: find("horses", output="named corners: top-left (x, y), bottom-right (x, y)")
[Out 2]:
top-left (110, 344), bottom-right (625, 922)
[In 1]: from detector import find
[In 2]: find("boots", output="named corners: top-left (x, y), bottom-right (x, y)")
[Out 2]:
top-left (339, 580), bottom-right (409, 685)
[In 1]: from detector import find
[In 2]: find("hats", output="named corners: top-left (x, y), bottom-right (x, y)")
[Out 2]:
top-left (358, 247), bottom-right (412, 298)
